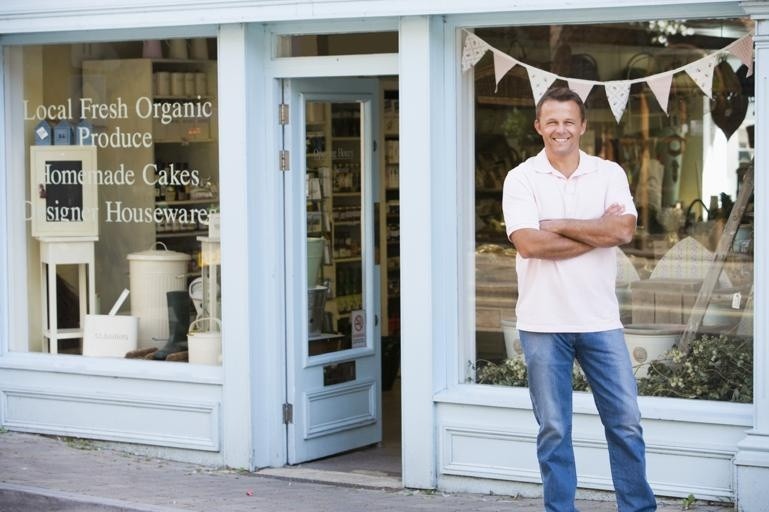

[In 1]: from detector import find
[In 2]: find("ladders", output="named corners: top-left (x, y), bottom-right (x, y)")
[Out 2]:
top-left (678, 160), bottom-right (755, 355)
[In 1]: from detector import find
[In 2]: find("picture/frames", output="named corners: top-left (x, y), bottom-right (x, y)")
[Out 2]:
top-left (29, 144), bottom-right (99, 238)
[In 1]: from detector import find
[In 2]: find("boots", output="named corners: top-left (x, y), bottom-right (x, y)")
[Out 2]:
top-left (152, 291), bottom-right (190, 361)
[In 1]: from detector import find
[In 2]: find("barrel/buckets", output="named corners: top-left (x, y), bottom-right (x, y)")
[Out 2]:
top-left (185, 319), bottom-right (223, 367)
top-left (126, 242), bottom-right (191, 345)
top-left (308, 284), bottom-right (328, 336)
top-left (307, 239), bottom-right (326, 286)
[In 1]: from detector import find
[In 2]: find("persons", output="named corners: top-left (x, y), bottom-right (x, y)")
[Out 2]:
top-left (500, 86), bottom-right (662, 512)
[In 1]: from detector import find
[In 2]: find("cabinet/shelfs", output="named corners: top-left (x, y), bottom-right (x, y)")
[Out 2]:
top-left (305, 75), bottom-right (398, 346)
top-left (81, 59), bottom-right (215, 320)
top-left (34, 237), bottom-right (100, 355)
